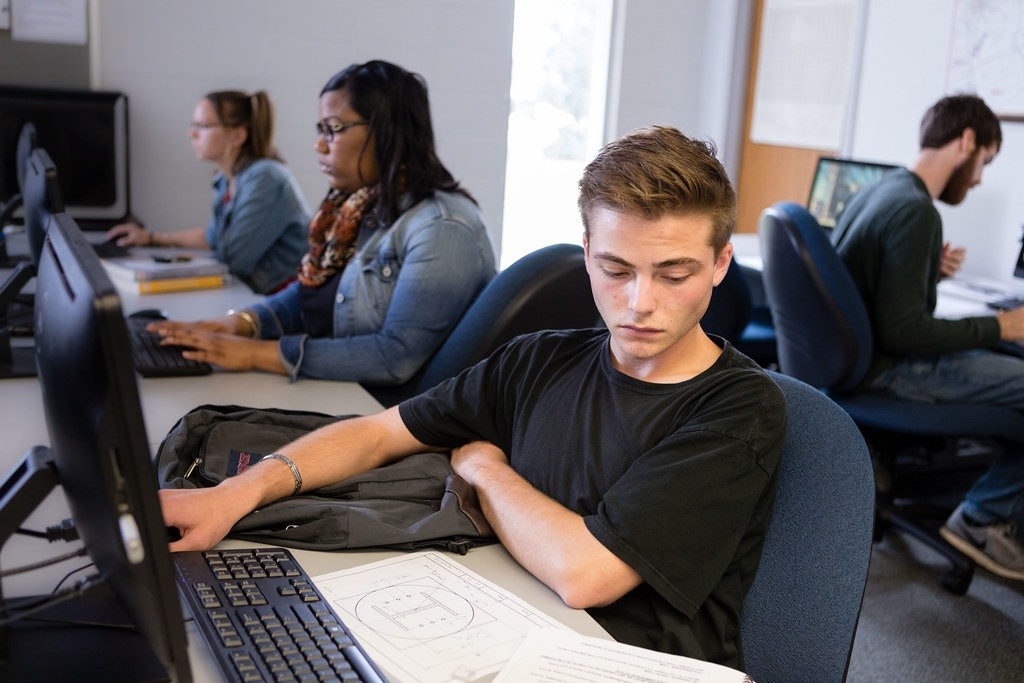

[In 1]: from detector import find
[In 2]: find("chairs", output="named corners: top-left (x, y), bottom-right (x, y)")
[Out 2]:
top-left (410, 200), bottom-right (979, 681)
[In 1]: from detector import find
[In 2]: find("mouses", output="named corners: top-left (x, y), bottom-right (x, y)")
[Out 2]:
top-left (166, 525), bottom-right (183, 542)
top-left (106, 232), bottom-right (128, 245)
top-left (129, 309), bottom-right (168, 319)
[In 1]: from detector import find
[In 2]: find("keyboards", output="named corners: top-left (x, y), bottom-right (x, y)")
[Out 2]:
top-left (126, 317), bottom-right (214, 377)
top-left (170, 548), bottom-right (387, 683)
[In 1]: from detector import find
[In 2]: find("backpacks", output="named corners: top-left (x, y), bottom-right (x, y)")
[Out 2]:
top-left (157, 402), bottom-right (493, 555)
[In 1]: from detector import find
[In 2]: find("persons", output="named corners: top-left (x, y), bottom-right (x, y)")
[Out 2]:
top-left (158, 123), bottom-right (788, 667)
top-left (105, 91), bottom-right (307, 294)
top-left (142, 59), bottom-right (498, 410)
top-left (828, 97), bottom-right (1024, 586)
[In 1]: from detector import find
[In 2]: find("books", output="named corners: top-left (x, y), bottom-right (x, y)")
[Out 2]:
top-left (101, 256), bottom-right (229, 293)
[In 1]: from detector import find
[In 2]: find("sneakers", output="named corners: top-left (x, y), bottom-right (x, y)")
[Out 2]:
top-left (941, 510), bottom-right (1024, 577)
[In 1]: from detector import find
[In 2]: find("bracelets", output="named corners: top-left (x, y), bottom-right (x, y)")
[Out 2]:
top-left (258, 453), bottom-right (302, 496)
top-left (227, 309), bottom-right (255, 337)
top-left (149, 231), bottom-right (155, 246)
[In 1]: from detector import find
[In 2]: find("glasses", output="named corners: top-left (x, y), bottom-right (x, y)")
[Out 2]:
top-left (190, 121), bottom-right (237, 130)
top-left (317, 115), bottom-right (371, 134)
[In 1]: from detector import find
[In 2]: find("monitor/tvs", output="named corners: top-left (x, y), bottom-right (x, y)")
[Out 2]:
top-left (0, 122), bottom-right (63, 379)
top-left (0, 212), bottom-right (194, 683)
top-left (804, 156), bottom-right (899, 232)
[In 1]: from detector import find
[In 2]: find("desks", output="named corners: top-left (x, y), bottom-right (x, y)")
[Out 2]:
top-left (726, 233), bottom-right (1024, 354)
top-left (0, 246), bottom-right (616, 683)
